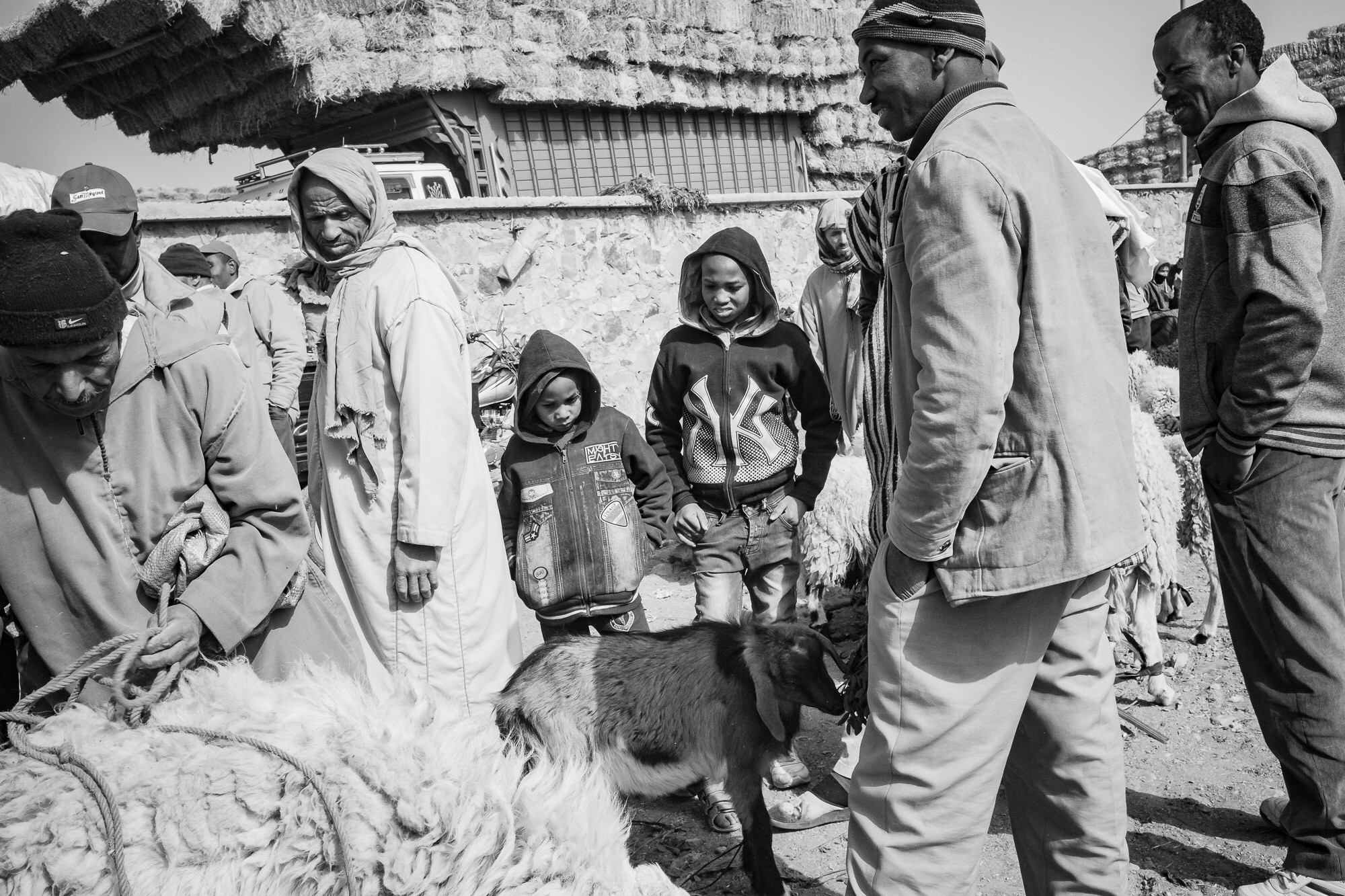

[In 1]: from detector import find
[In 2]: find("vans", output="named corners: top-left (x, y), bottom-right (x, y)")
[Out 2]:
top-left (219, 144), bottom-right (463, 202)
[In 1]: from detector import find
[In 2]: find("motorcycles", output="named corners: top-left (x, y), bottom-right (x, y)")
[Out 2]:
top-left (465, 306), bottom-right (532, 467)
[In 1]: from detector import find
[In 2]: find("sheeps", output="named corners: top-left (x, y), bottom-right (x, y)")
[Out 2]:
top-left (0, 643), bottom-right (692, 895)
top-left (493, 606), bottom-right (854, 896)
top-left (790, 350), bottom-right (1222, 705)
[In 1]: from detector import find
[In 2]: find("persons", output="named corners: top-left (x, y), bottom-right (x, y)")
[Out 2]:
top-left (1151, 0), bottom-right (1345, 896)
top-left (51, 163), bottom-right (308, 476)
top-left (500, 330), bottom-right (744, 834)
top-left (645, 227), bottom-right (840, 789)
top-left (1117, 256), bottom-right (1184, 355)
top-left (287, 146), bottom-right (544, 720)
top-left (767, 0), bottom-right (1150, 896)
top-left (0, 209), bottom-right (379, 723)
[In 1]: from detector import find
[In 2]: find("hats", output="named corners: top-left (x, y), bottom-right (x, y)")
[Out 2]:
top-left (198, 241), bottom-right (240, 267)
top-left (0, 207), bottom-right (128, 348)
top-left (851, 0), bottom-right (986, 63)
top-left (51, 164), bottom-right (139, 237)
top-left (159, 241), bottom-right (212, 278)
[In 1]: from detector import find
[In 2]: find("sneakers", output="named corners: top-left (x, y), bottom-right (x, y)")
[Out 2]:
top-left (1235, 867), bottom-right (1345, 896)
top-left (1260, 796), bottom-right (1289, 828)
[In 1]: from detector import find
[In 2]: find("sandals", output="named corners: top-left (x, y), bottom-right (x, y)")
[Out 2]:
top-left (698, 781), bottom-right (741, 832)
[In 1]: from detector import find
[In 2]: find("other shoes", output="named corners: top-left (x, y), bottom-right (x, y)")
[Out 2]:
top-left (769, 747), bottom-right (811, 789)
top-left (768, 799), bottom-right (850, 831)
